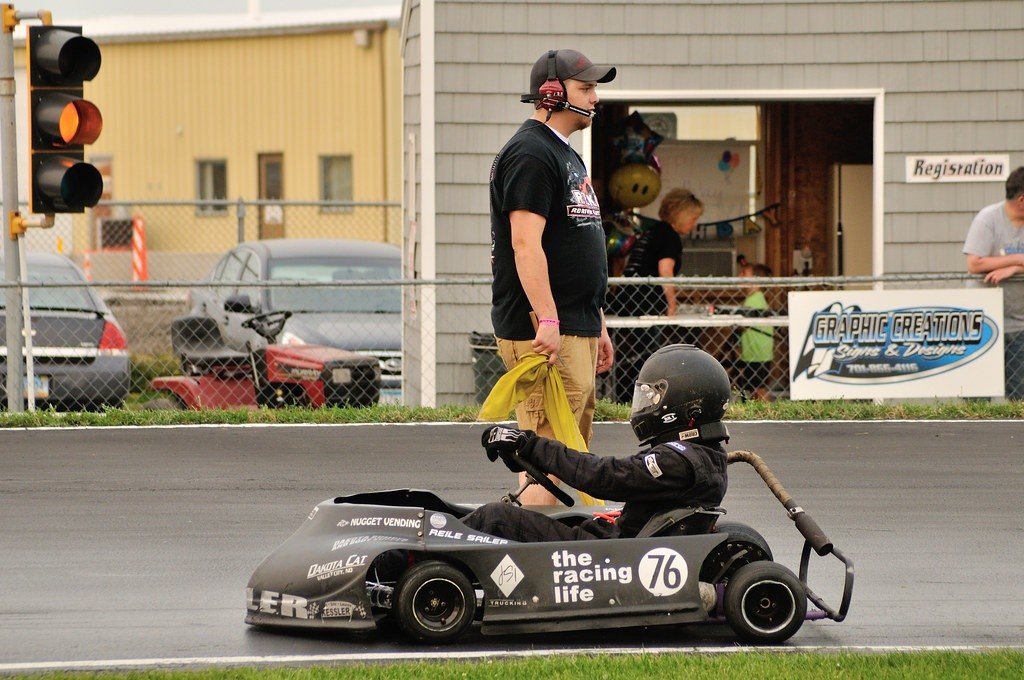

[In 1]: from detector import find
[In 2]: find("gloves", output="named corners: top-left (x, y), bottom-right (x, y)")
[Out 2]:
top-left (482, 425), bottom-right (536, 462)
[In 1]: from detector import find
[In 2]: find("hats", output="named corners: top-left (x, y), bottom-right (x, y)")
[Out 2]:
top-left (530, 49), bottom-right (616, 95)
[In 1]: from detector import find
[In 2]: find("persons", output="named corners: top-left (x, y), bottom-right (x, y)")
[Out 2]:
top-left (459, 344), bottom-right (732, 543)
top-left (612, 188), bottom-right (705, 405)
top-left (962, 166), bottom-right (1024, 403)
top-left (737, 263), bottom-right (776, 405)
top-left (489, 49), bottom-right (616, 506)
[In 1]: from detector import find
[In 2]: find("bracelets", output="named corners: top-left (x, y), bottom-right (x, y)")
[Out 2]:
top-left (537, 319), bottom-right (561, 325)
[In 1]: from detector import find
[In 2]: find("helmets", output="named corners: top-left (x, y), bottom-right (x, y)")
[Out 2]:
top-left (630, 344), bottom-right (731, 447)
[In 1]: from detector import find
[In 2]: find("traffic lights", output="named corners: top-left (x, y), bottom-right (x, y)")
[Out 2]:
top-left (26, 25), bottom-right (102, 214)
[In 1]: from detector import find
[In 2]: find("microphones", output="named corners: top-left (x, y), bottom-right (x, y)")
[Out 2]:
top-left (563, 102), bottom-right (595, 118)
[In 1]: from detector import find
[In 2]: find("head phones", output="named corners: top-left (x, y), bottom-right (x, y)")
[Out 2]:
top-left (539, 49), bottom-right (568, 112)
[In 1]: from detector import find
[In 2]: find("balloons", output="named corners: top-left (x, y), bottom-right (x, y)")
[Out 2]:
top-left (598, 111), bottom-right (664, 277)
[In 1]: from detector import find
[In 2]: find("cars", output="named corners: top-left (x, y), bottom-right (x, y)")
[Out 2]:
top-left (0, 251), bottom-right (129, 415)
top-left (189, 238), bottom-right (402, 388)
